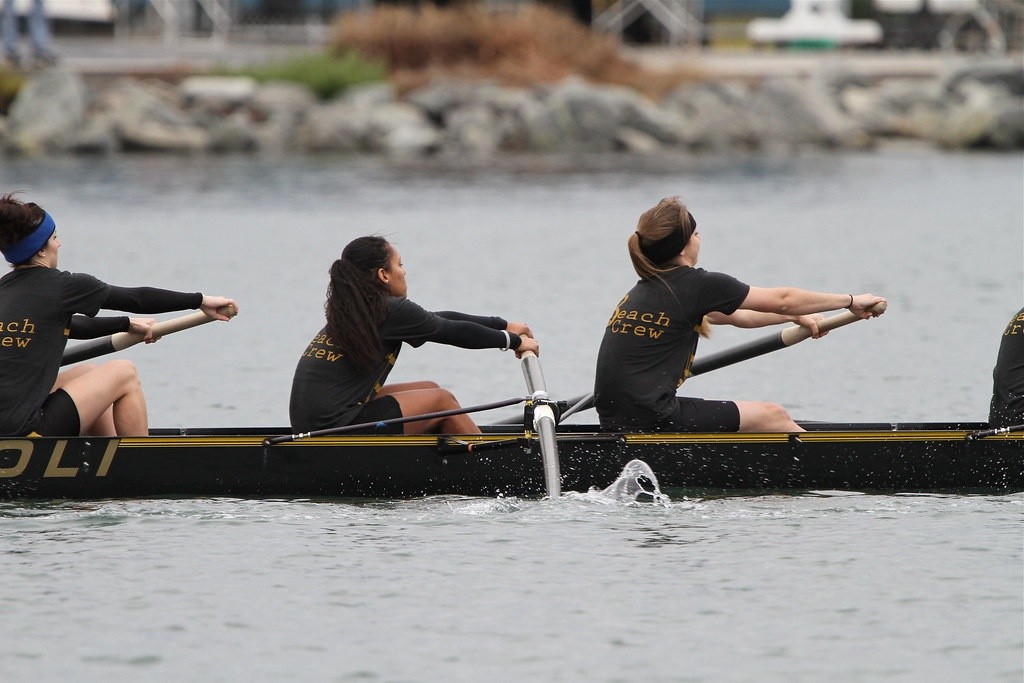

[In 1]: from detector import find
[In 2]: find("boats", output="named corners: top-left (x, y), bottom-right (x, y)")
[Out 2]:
top-left (0, 422), bottom-right (1024, 496)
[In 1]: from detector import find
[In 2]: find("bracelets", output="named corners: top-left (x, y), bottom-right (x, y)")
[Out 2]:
top-left (500, 330), bottom-right (510, 352)
top-left (845, 294), bottom-right (854, 309)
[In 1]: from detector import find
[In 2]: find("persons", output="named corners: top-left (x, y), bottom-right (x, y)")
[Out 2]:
top-left (288, 236), bottom-right (540, 436)
top-left (0, 0), bottom-right (57, 68)
top-left (988, 307), bottom-right (1024, 431)
top-left (0, 198), bottom-right (239, 437)
top-left (594, 196), bottom-right (887, 432)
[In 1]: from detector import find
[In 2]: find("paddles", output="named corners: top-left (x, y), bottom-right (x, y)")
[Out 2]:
top-left (60, 304), bottom-right (235, 367)
top-left (494, 302), bottom-right (888, 424)
top-left (520, 333), bottom-right (561, 501)
